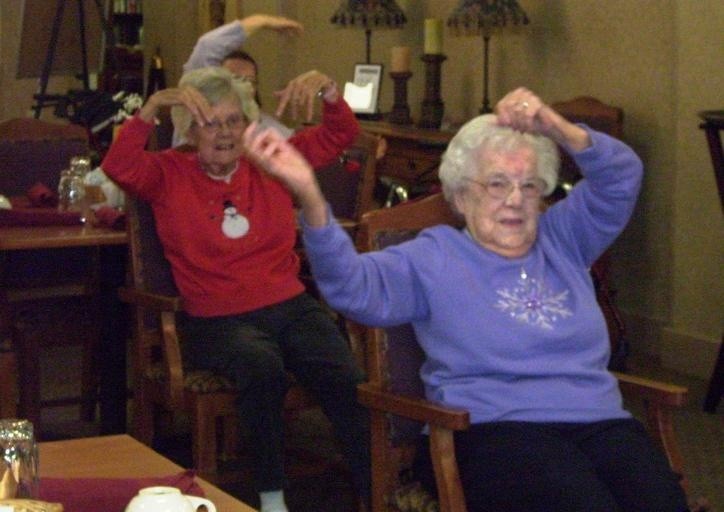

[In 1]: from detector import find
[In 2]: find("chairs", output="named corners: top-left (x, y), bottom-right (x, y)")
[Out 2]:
top-left (315, 134), bottom-right (378, 254)
top-left (358, 194), bottom-right (688, 512)
top-left (118, 195), bottom-right (366, 486)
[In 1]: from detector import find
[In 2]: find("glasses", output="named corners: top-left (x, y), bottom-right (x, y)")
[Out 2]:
top-left (467, 176), bottom-right (543, 200)
top-left (204, 115), bottom-right (242, 133)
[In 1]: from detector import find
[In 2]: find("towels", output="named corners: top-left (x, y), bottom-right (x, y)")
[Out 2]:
top-left (1, 208), bottom-right (83, 226)
top-left (27, 182), bottom-right (59, 208)
top-left (95, 206), bottom-right (127, 231)
top-left (38, 470), bottom-right (206, 512)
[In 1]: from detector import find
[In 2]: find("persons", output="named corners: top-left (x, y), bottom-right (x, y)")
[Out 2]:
top-left (174, 13), bottom-right (302, 152)
top-left (240, 85), bottom-right (703, 510)
top-left (99, 68), bottom-right (383, 512)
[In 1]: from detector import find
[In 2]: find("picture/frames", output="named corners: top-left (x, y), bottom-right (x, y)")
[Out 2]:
top-left (351, 64), bottom-right (382, 116)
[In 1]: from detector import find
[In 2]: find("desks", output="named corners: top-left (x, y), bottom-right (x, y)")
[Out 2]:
top-left (0, 208), bottom-right (357, 434)
top-left (0, 434), bottom-right (259, 512)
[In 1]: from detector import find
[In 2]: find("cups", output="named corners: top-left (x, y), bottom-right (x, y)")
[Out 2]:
top-left (124, 484), bottom-right (218, 512)
top-left (2, 415), bottom-right (40, 505)
top-left (58, 167), bottom-right (85, 212)
top-left (71, 153), bottom-right (92, 178)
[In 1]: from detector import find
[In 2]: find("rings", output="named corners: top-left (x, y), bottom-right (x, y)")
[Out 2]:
top-left (523, 101), bottom-right (529, 108)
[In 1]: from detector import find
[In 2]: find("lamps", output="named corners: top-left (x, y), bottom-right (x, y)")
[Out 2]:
top-left (447, 1), bottom-right (531, 114)
top-left (329, 0), bottom-right (405, 63)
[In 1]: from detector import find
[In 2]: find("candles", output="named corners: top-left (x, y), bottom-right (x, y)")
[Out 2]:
top-left (424, 19), bottom-right (443, 55)
top-left (392, 45), bottom-right (409, 72)
